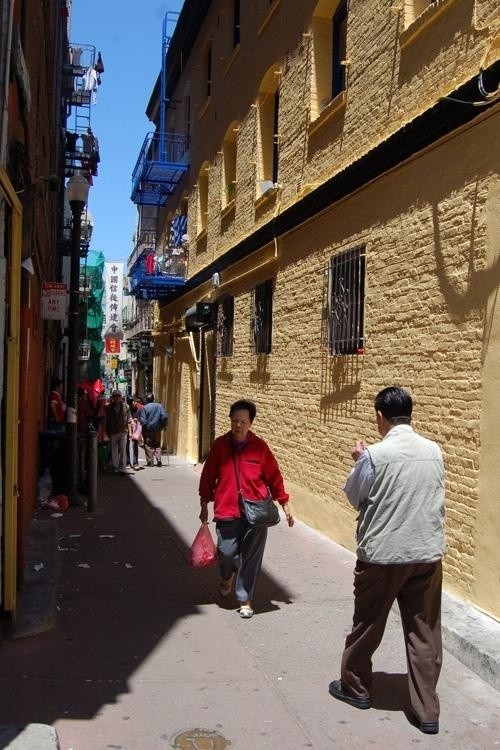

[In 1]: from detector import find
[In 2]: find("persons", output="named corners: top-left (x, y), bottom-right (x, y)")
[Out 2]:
top-left (198, 398), bottom-right (295, 618)
top-left (47, 377), bottom-right (168, 474)
top-left (329, 387), bottom-right (447, 734)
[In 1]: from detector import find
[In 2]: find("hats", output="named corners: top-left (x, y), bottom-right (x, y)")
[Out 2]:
top-left (111, 389), bottom-right (123, 398)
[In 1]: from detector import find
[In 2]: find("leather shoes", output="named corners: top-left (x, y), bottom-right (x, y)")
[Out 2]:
top-left (419, 720), bottom-right (441, 735)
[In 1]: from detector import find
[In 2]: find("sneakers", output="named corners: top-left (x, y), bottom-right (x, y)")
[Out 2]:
top-left (125, 458), bottom-right (163, 470)
top-left (329, 680), bottom-right (371, 709)
top-left (219, 571), bottom-right (237, 597)
top-left (240, 602), bottom-right (253, 618)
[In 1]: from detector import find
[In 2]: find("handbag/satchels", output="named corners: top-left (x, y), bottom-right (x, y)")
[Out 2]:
top-left (237, 489), bottom-right (282, 529)
